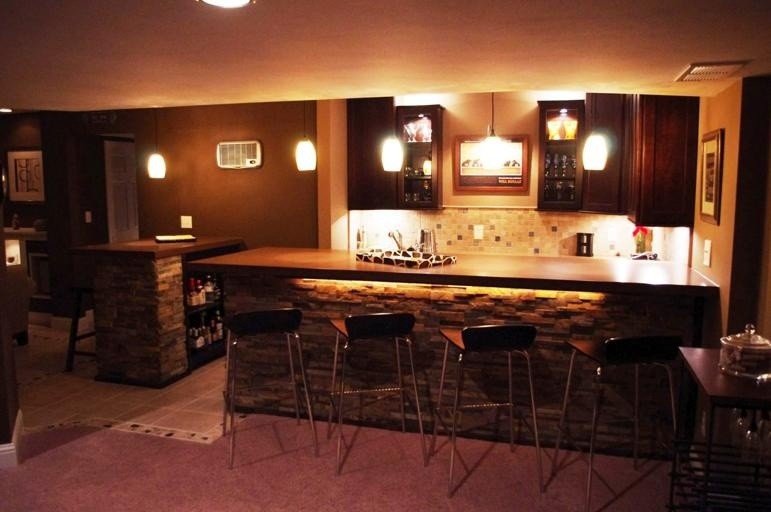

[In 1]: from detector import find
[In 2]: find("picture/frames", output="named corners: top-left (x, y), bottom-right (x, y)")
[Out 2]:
top-left (6, 145), bottom-right (43, 205)
top-left (452, 135), bottom-right (530, 195)
top-left (700, 128), bottom-right (724, 226)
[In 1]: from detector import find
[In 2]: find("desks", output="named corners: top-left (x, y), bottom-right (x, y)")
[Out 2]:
top-left (668, 346), bottom-right (771, 512)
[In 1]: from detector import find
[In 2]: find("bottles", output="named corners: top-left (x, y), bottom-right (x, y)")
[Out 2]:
top-left (728, 408), bottom-right (771, 482)
top-left (545, 152), bottom-right (576, 200)
top-left (185, 274), bottom-right (223, 349)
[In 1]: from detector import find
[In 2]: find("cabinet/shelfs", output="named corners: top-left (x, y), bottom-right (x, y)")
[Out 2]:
top-left (535, 100), bottom-right (583, 211)
top-left (628, 95), bottom-right (699, 228)
top-left (580, 93), bottom-right (630, 213)
top-left (182, 245), bottom-right (242, 369)
top-left (346, 98), bottom-right (443, 210)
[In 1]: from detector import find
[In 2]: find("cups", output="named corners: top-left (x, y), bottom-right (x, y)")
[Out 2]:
top-left (405, 193), bottom-right (411, 202)
top-left (413, 192), bottom-right (420, 203)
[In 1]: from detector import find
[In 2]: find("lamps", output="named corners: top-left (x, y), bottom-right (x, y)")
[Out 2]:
top-left (380, 96), bottom-right (403, 172)
top-left (295, 101), bottom-right (317, 171)
top-left (147, 109), bottom-right (166, 178)
top-left (484, 92), bottom-right (502, 149)
top-left (582, 92), bottom-right (609, 170)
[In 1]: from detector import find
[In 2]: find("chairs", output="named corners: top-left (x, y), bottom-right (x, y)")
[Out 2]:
top-left (426, 323), bottom-right (544, 497)
top-left (220, 308), bottom-right (319, 469)
top-left (327, 311), bottom-right (425, 474)
top-left (63, 237), bottom-right (96, 372)
top-left (552, 333), bottom-right (680, 512)
